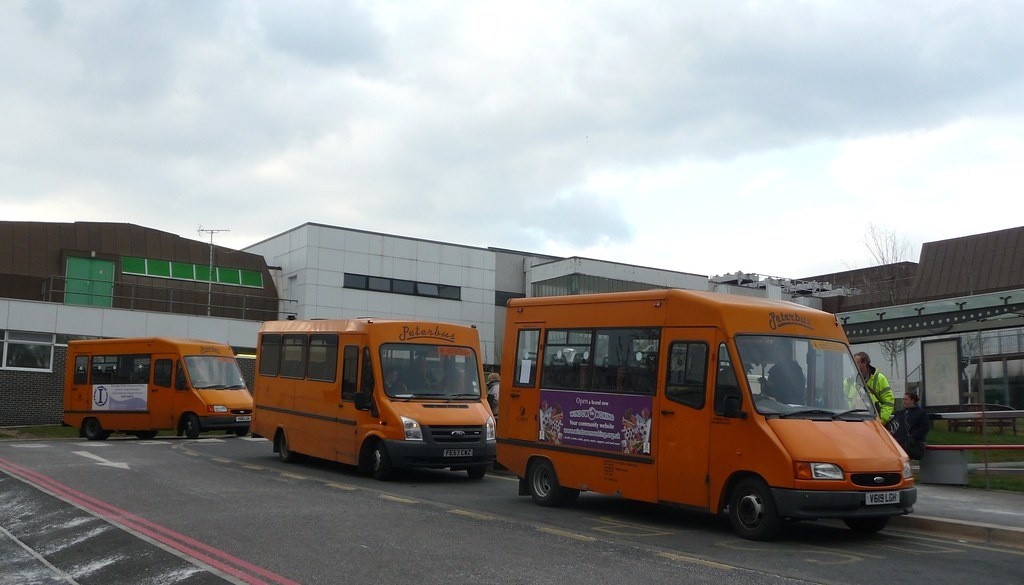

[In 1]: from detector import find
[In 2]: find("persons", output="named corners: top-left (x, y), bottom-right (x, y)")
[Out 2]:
top-left (384, 366), bottom-right (407, 395)
top-left (439, 356), bottom-right (464, 393)
top-left (637, 352), bottom-right (670, 397)
top-left (410, 358), bottom-right (439, 388)
top-left (758, 342), bottom-right (806, 405)
top-left (892, 392), bottom-right (931, 485)
top-left (717, 353), bottom-right (754, 401)
top-left (486, 373), bottom-right (500, 427)
top-left (843, 352), bottom-right (894, 427)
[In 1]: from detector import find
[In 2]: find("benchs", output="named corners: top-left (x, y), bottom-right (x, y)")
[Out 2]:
top-left (946, 403), bottom-right (1017, 436)
top-left (890, 410), bottom-right (1024, 485)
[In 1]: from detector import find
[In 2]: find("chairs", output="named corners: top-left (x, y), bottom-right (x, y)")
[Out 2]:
top-left (283, 360), bottom-right (336, 379)
top-left (520, 352), bottom-right (653, 393)
top-left (76, 365), bottom-right (117, 384)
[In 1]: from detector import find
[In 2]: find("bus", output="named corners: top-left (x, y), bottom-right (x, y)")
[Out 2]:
top-left (251, 316), bottom-right (497, 481)
top-left (62, 337), bottom-right (254, 441)
top-left (496, 288), bottom-right (917, 542)
